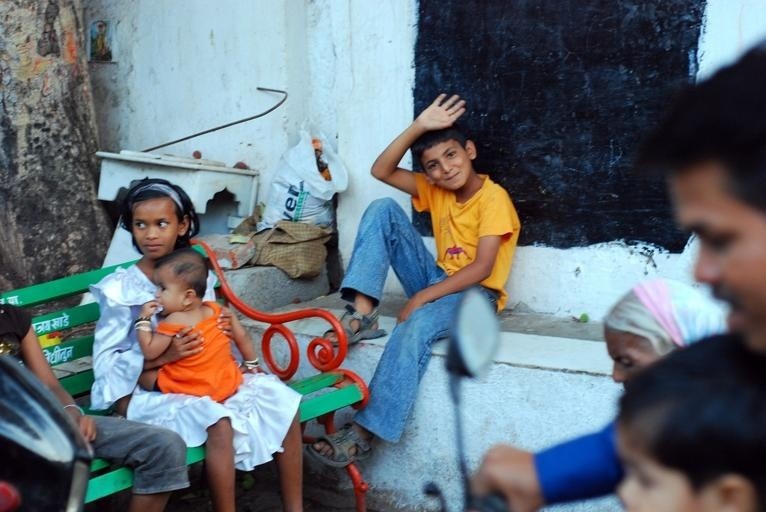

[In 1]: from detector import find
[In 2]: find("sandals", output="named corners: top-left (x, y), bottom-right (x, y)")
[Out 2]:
top-left (322, 304), bottom-right (385, 347)
top-left (306, 423), bottom-right (373, 467)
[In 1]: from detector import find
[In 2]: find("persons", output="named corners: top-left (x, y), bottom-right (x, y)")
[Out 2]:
top-left (87, 179), bottom-right (304, 510)
top-left (115, 247), bottom-right (268, 420)
top-left (305, 94), bottom-right (520, 468)
top-left (468, 36), bottom-right (765, 510)
top-left (1, 304), bottom-right (191, 512)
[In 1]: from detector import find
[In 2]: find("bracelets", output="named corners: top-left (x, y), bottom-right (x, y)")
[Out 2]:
top-left (62, 404), bottom-right (85, 416)
top-left (133, 316), bottom-right (153, 333)
top-left (241, 357), bottom-right (261, 369)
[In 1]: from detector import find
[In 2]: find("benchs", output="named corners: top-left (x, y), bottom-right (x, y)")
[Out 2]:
top-left (0, 240), bottom-right (370, 512)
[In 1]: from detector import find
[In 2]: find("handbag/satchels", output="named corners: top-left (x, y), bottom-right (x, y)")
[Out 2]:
top-left (233, 207), bottom-right (333, 280)
